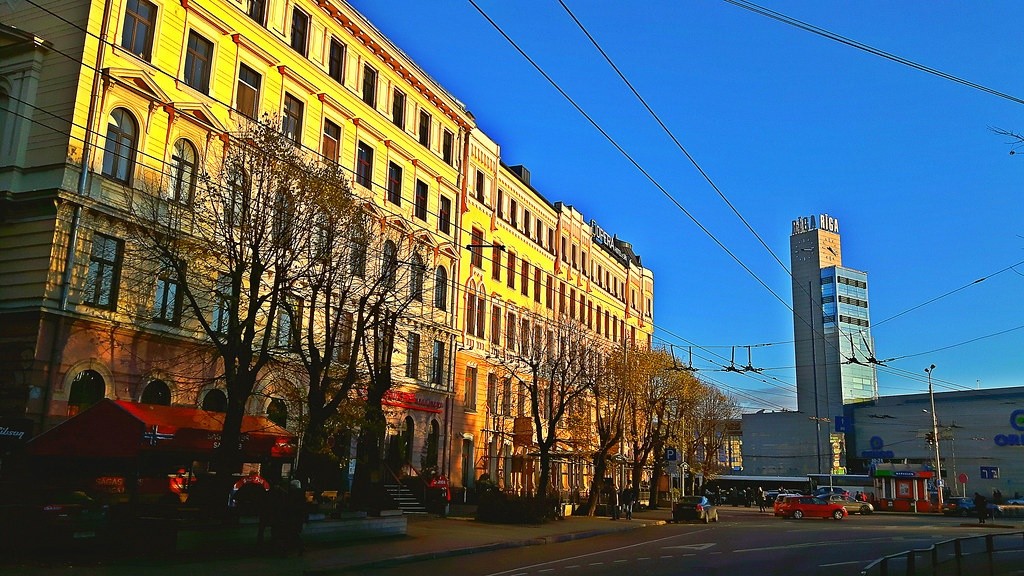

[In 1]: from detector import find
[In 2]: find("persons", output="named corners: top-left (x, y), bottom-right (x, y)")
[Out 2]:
top-left (278, 480), bottom-right (306, 559)
top-left (622, 483), bottom-right (636, 520)
top-left (713, 484), bottom-right (876, 515)
top-left (993, 489), bottom-right (1003, 505)
top-left (973, 492), bottom-right (987, 523)
top-left (608, 484), bottom-right (622, 520)
top-left (942, 486), bottom-right (952, 501)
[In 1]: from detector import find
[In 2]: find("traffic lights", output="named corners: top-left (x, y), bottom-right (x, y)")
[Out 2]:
top-left (925, 431), bottom-right (934, 446)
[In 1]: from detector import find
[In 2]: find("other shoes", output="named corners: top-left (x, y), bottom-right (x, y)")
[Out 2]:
top-left (616, 518), bottom-right (619, 519)
top-left (279, 542), bottom-right (289, 560)
top-left (271, 543), bottom-right (279, 555)
top-left (629, 516), bottom-right (631, 520)
top-left (610, 519), bottom-right (616, 520)
top-left (248, 550), bottom-right (265, 557)
top-left (296, 546), bottom-right (308, 556)
top-left (626, 517), bottom-right (628, 520)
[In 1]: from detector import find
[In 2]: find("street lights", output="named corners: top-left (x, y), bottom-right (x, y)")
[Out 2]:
top-left (923, 365), bottom-right (943, 512)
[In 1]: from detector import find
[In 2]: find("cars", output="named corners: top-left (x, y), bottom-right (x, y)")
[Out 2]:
top-left (774, 493), bottom-right (799, 516)
top-left (817, 492), bottom-right (874, 516)
top-left (672, 494), bottom-right (720, 525)
top-left (780, 494), bottom-right (849, 520)
top-left (940, 495), bottom-right (1003, 519)
top-left (1007, 496), bottom-right (1024, 505)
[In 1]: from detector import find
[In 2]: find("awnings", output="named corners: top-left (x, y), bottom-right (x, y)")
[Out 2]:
top-left (22, 398), bottom-right (300, 495)
